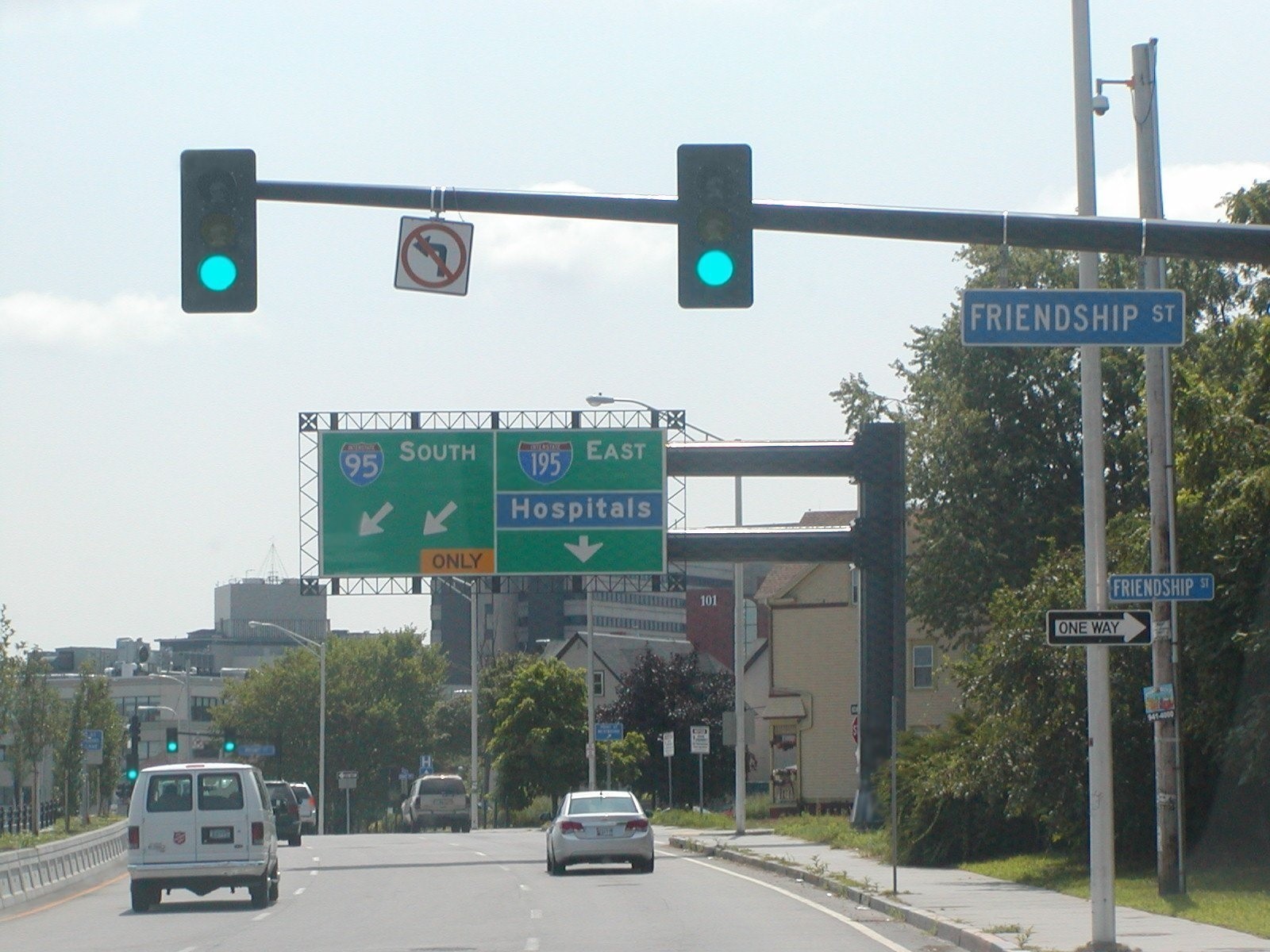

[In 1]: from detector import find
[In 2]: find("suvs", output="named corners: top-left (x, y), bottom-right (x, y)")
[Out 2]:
top-left (290, 780), bottom-right (317, 833)
top-left (125, 763), bottom-right (283, 911)
top-left (397, 774), bottom-right (473, 832)
top-left (263, 777), bottom-right (303, 848)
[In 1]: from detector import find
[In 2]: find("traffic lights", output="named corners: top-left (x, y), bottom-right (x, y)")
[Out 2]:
top-left (676, 142), bottom-right (753, 312)
top-left (164, 727), bottom-right (178, 754)
top-left (224, 726), bottom-right (238, 752)
top-left (179, 147), bottom-right (256, 313)
top-left (127, 754), bottom-right (139, 781)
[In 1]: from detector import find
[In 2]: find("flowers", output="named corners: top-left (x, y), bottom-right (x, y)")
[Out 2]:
top-left (777, 739), bottom-right (797, 752)
top-left (771, 768), bottom-right (798, 786)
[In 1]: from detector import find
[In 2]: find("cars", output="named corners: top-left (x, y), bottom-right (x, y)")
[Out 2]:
top-left (540, 790), bottom-right (656, 876)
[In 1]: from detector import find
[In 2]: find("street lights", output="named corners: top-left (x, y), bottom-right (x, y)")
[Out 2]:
top-left (1095, 34), bottom-right (1189, 898)
top-left (583, 393), bottom-right (748, 836)
top-left (147, 657), bottom-right (194, 764)
top-left (247, 619), bottom-right (327, 836)
top-left (139, 705), bottom-right (179, 763)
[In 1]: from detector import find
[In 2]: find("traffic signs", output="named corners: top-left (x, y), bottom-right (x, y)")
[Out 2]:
top-left (311, 425), bottom-right (673, 577)
top-left (594, 723), bottom-right (624, 742)
top-left (1043, 609), bottom-right (1157, 646)
